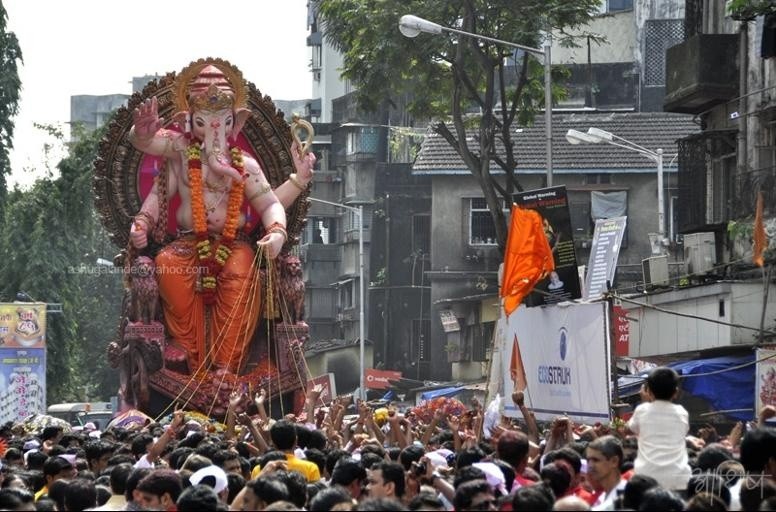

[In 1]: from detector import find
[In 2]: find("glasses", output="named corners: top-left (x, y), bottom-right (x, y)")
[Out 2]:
top-left (464, 500), bottom-right (502, 511)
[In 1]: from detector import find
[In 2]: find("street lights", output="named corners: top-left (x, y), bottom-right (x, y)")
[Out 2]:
top-left (396, 11), bottom-right (556, 189)
top-left (15, 288), bottom-right (39, 303)
top-left (563, 125), bottom-right (667, 256)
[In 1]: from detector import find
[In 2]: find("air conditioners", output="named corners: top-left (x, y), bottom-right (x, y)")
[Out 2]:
top-left (683, 232), bottom-right (716, 275)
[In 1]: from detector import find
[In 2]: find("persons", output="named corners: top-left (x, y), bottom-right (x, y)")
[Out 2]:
top-left (547, 270), bottom-right (563, 290)
top-left (1, 382), bottom-right (776, 512)
top-left (625, 369), bottom-right (692, 487)
top-left (543, 218), bottom-right (557, 250)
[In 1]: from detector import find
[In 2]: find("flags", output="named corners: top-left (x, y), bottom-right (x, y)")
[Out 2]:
top-left (500, 204), bottom-right (557, 320)
top-left (511, 334), bottom-right (527, 392)
top-left (752, 193), bottom-right (767, 267)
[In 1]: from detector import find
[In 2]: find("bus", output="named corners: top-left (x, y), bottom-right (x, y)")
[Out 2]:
top-left (46, 402), bottom-right (113, 431)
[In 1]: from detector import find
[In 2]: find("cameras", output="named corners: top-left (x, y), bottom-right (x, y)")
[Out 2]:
top-left (411, 457), bottom-right (426, 475)
